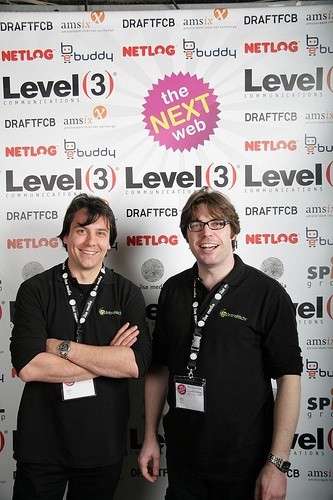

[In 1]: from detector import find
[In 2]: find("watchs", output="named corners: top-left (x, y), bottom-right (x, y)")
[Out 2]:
top-left (58, 339), bottom-right (72, 360)
top-left (265, 452), bottom-right (293, 473)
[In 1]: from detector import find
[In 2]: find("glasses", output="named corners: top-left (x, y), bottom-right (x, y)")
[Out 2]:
top-left (184, 219), bottom-right (230, 232)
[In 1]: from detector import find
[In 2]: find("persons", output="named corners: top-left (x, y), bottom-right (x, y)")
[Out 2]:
top-left (8, 192), bottom-right (153, 500)
top-left (134, 183), bottom-right (304, 500)
